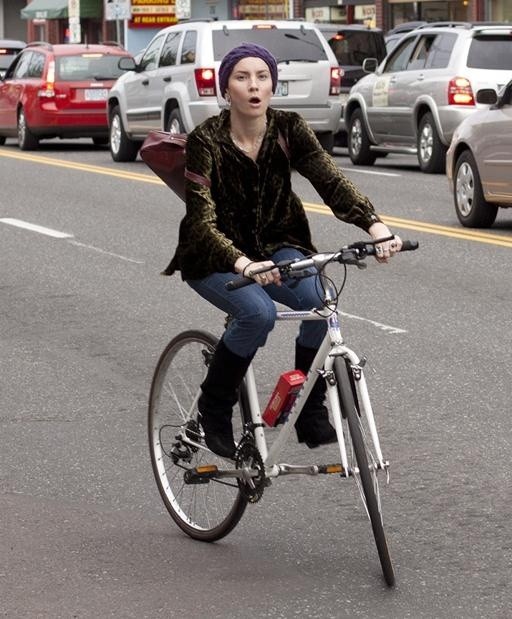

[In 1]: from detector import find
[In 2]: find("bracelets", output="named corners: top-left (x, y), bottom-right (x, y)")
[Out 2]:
top-left (242, 260), bottom-right (256, 278)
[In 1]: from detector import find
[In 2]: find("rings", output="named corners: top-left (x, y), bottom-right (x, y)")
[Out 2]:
top-left (375, 246), bottom-right (382, 253)
top-left (382, 247), bottom-right (388, 252)
top-left (260, 275), bottom-right (268, 283)
top-left (390, 242), bottom-right (397, 248)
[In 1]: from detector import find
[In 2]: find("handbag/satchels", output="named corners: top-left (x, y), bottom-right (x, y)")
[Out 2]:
top-left (136, 126), bottom-right (216, 205)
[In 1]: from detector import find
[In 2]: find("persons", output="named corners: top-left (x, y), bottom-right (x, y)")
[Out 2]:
top-left (162, 42), bottom-right (402, 460)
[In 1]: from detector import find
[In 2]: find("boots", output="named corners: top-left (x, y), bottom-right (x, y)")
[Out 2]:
top-left (197, 332), bottom-right (258, 459)
top-left (289, 334), bottom-right (347, 450)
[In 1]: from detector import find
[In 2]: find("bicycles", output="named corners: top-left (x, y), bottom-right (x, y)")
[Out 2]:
top-left (146, 233), bottom-right (419, 587)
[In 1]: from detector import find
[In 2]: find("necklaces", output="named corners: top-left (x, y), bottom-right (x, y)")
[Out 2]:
top-left (231, 111), bottom-right (267, 153)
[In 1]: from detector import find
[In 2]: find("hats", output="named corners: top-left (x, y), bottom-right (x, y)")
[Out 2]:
top-left (219, 42), bottom-right (279, 99)
